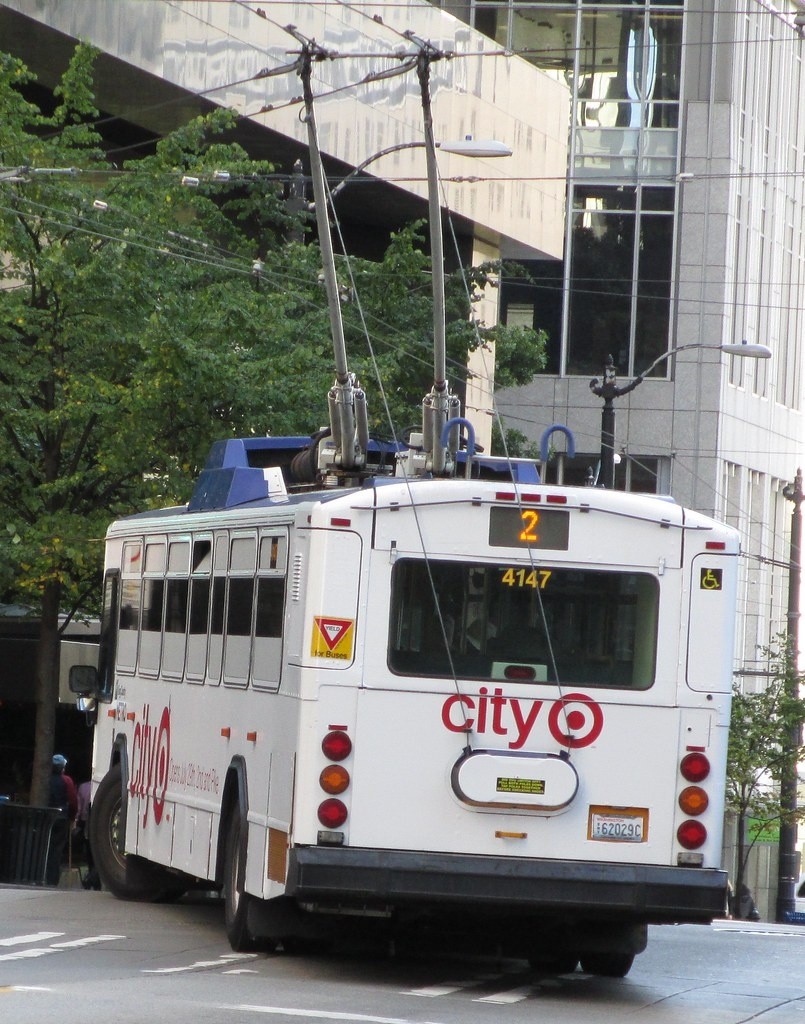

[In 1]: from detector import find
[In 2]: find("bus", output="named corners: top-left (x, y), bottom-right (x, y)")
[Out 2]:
top-left (68, 48), bottom-right (748, 979)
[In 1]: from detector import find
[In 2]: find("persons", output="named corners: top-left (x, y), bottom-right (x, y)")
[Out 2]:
top-left (484, 602), bottom-right (557, 683)
top-left (72, 768), bottom-right (102, 891)
top-left (43, 764), bottom-right (72, 888)
top-left (452, 606), bottom-right (498, 677)
top-left (420, 602), bottom-right (466, 676)
top-left (51, 754), bottom-right (78, 867)
top-left (732, 884), bottom-right (760, 921)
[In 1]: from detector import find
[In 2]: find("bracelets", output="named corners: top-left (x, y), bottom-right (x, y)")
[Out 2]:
top-left (74, 818), bottom-right (78, 821)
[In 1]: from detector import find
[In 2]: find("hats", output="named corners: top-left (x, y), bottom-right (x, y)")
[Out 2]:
top-left (52, 755), bottom-right (67, 765)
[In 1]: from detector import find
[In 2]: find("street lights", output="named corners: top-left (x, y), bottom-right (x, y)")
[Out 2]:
top-left (589, 339), bottom-right (774, 493)
